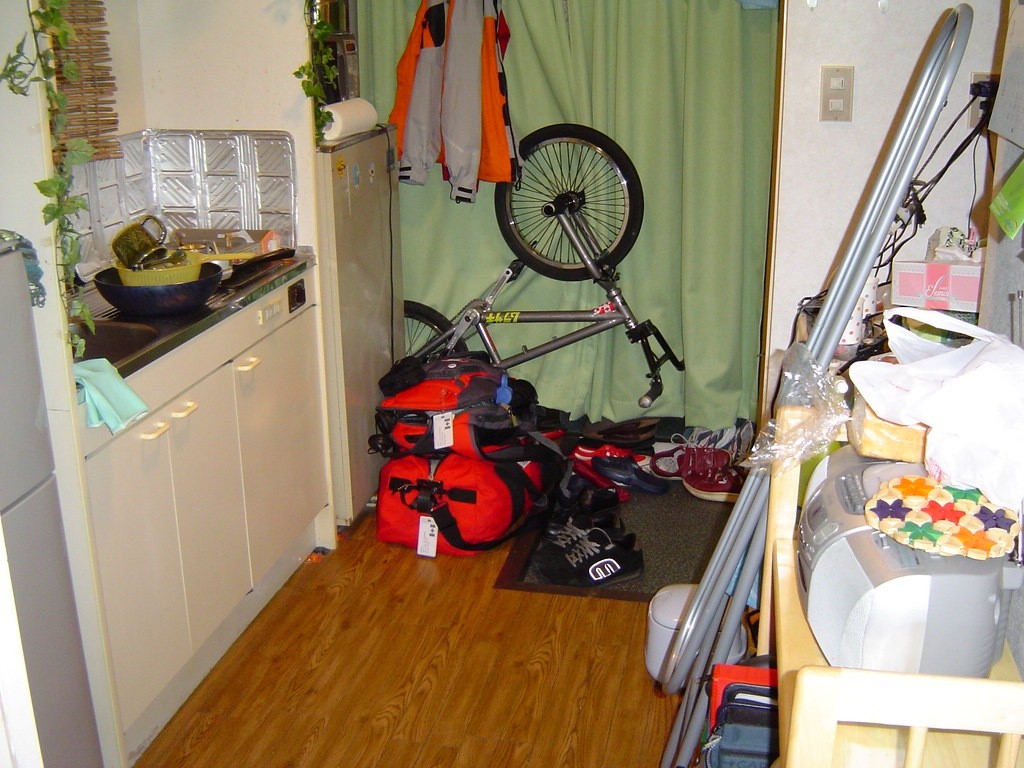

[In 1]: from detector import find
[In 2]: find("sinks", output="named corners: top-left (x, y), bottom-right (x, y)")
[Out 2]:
top-left (69, 322), bottom-right (160, 364)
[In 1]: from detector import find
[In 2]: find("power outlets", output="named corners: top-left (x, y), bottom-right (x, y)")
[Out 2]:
top-left (967, 71), bottom-right (1001, 131)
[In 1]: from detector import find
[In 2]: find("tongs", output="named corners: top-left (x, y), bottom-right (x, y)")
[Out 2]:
top-left (132, 250), bottom-right (186, 272)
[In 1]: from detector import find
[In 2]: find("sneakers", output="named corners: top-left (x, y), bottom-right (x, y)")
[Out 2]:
top-left (573, 447), bottom-right (652, 469)
top-left (531, 507), bottom-right (623, 568)
top-left (651, 434), bottom-right (732, 480)
top-left (683, 463), bottom-right (748, 504)
top-left (541, 529), bottom-right (644, 586)
top-left (687, 425), bottom-right (754, 464)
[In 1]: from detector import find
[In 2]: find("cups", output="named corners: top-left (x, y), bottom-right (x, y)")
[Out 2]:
top-left (111, 214), bottom-right (168, 265)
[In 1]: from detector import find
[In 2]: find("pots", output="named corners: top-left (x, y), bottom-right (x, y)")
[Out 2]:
top-left (93, 247), bottom-right (296, 319)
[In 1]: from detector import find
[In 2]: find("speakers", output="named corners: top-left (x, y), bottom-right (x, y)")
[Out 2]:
top-left (798, 443), bottom-right (1022, 678)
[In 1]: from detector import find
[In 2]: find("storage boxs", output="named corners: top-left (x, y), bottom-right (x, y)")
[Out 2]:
top-left (889, 260), bottom-right (986, 315)
top-left (846, 349), bottom-right (930, 466)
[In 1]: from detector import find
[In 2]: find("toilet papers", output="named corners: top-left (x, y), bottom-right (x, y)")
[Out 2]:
top-left (318, 98), bottom-right (378, 142)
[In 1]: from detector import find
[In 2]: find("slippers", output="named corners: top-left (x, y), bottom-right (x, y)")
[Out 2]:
top-left (590, 453), bottom-right (669, 494)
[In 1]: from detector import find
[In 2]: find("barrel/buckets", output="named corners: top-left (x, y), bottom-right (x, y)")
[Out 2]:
top-left (644, 582), bottom-right (749, 690)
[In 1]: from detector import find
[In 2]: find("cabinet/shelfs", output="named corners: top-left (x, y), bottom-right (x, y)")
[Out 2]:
top-left (76, 264), bottom-right (338, 768)
top-left (755, 404), bottom-right (1024, 768)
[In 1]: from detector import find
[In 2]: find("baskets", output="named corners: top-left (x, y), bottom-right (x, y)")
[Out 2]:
top-left (113, 249), bottom-right (200, 285)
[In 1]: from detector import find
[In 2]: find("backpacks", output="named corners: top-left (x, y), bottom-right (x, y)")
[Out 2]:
top-left (372, 352), bottom-right (547, 463)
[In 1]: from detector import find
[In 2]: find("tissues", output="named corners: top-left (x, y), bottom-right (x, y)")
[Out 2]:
top-left (890, 246), bottom-right (983, 314)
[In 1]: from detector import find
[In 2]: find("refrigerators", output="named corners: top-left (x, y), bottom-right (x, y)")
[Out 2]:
top-left (0, 226), bottom-right (109, 768)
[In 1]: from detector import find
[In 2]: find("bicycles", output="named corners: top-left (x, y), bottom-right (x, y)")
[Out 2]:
top-left (398, 124), bottom-right (684, 407)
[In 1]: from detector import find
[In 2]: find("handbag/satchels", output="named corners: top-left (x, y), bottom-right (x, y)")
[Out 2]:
top-left (849, 308), bottom-right (1023, 513)
top-left (376, 450), bottom-right (560, 554)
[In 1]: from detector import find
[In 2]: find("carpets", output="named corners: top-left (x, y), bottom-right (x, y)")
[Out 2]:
top-left (494, 474), bottom-right (736, 603)
